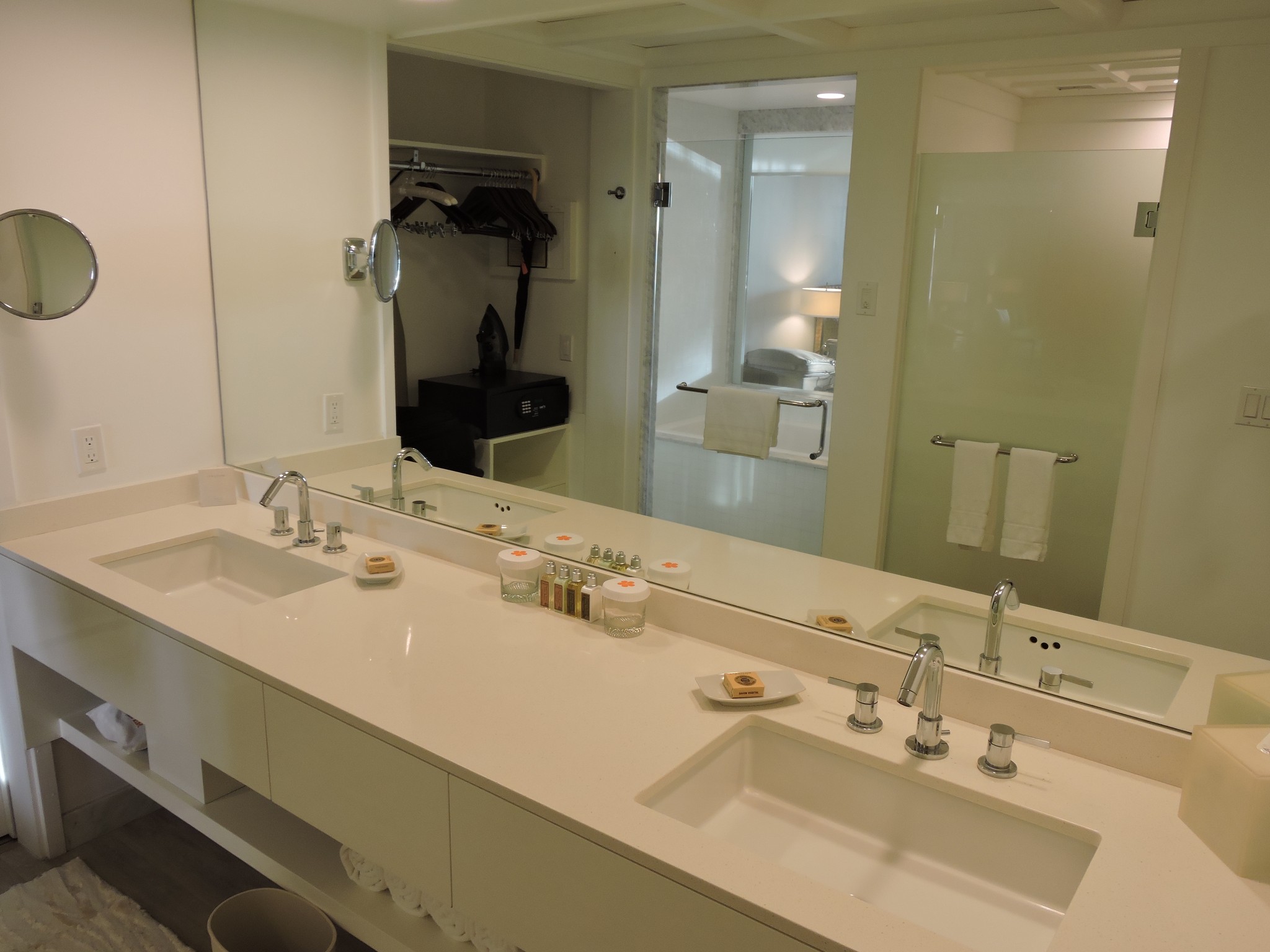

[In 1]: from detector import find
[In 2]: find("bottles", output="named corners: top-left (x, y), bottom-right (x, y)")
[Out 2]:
top-left (540, 561), bottom-right (603, 623)
top-left (586, 544), bottom-right (644, 578)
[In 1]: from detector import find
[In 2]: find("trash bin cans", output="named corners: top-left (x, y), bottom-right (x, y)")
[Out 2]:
top-left (208, 888), bottom-right (337, 952)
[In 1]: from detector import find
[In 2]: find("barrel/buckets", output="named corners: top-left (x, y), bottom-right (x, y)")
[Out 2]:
top-left (207, 888), bottom-right (337, 952)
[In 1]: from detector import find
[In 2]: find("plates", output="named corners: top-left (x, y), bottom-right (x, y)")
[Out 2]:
top-left (807, 607), bottom-right (868, 639)
top-left (494, 525), bottom-right (527, 540)
top-left (694, 670), bottom-right (807, 706)
top-left (354, 551), bottom-right (403, 583)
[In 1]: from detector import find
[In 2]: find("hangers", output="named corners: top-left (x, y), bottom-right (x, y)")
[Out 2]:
top-left (390, 166), bottom-right (559, 244)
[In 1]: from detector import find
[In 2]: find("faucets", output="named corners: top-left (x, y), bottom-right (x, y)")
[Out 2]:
top-left (388, 447), bottom-right (433, 513)
top-left (976, 578), bottom-right (1022, 682)
top-left (896, 644), bottom-right (957, 761)
top-left (258, 470), bottom-right (326, 549)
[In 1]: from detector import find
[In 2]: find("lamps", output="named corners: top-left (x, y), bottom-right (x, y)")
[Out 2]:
top-left (797, 282), bottom-right (842, 320)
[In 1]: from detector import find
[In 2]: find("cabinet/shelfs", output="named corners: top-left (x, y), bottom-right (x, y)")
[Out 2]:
top-left (480, 423), bottom-right (571, 499)
top-left (0, 556), bottom-right (808, 950)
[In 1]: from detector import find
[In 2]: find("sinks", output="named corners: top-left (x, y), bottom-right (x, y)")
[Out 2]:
top-left (635, 713), bottom-right (1106, 952)
top-left (88, 527), bottom-right (350, 619)
top-left (864, 595), bottom-right (1198, 722)
top-left (356, 476), bottom-right (569, 541)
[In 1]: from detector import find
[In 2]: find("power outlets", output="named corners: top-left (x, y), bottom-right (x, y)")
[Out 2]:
top-left (855, 281), bottom-right (877, 317)
top-left (1234, 385), bottom-right (1270, 429)
top-left (324, 393), bottom-right (345, 434)
top-left (74, 424), bottom-right (108, 476)
top-left (558, 332), bottom-right (573, 362)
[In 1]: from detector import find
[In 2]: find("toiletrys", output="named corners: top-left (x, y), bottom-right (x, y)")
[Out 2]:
top-left (579, 572), bottom-right (603, 624)
top-left (597, 547), bottom-right (615, 571)
top-left (553, 564), bottom-right (571, 615)
top-left (538, 560), bottom-right (561, 612)
top-left (584, 544), bottom-right (602, 567)
top-left (611, 551), bottom-right (632, 575)
top-left (627, 555), bottom-right (645, 578)
top-left (566, 566), bottom-right (586, 620)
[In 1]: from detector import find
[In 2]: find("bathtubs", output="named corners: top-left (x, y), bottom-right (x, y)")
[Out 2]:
top-left (654, 408), bottom-right (832, 557)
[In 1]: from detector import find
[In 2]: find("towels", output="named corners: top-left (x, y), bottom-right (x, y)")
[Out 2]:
top-left (998, 447), bottom-right (1059, 563)
top-left (336, 844), bottom-right (396, 896)
top-left (700, 386), bottom-right (785, 461)
top-left (426, 899), bottom-right (473, 943)
top-left (379, 865), bottom-right (436, 921)
top-left (946, 438), bottom-right (1001, 554)
top-left (468, 932), bottom-right (520, 952)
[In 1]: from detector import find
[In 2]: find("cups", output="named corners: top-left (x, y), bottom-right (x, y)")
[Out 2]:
top-left (649, 560), bottom-right (690, 590)
top-left (603, 577), bottom-right (647, 638)
top-left (544, 533), bottom-right (585, 560)
top-left (499, 548), bottom-right (540, 603)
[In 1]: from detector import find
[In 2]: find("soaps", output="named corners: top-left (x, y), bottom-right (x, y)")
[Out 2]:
top-left (473, 521), bottom-right (505, 537)
top-left (722, 670), bottom-right (767, 701)
top-left (816, 612), bottom-right (853, 634)
top-left (365, 554), bottom-right (396, 574)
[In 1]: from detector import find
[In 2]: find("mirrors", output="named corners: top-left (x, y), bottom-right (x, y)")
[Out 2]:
top-left (343, 220), bottom-right (402, 304)
top-left (0, 208), bottom-right (99, 321)
top-left (190, 0), bottom-right (1270, 757)
